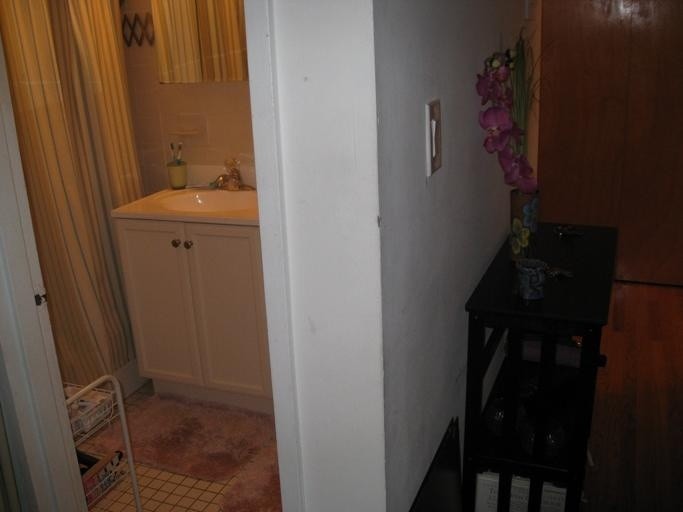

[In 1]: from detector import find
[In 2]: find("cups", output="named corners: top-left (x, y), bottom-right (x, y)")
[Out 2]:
top-left (514, 258), bottom-right (550, 302)
top-left (166, 161), bottom-right (189, 190)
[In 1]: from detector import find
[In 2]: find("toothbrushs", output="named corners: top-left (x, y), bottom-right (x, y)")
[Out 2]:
top-left (167, 142), bottom-right (178, 164)
top-left (176, 141), bottom-right (183, 166)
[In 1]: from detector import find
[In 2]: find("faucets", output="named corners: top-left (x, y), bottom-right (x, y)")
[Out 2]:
top-left (214, 167), bottom-right (240, 191)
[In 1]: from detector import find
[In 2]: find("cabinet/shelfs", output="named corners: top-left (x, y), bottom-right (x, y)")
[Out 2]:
top-left (107, 219), bottom-right (273, 420)
top-left (60, 374), bottom-right (143, 512)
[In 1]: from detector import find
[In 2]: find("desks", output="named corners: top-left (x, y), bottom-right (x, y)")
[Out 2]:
top-left (463, 220), bottom-right (621, 511)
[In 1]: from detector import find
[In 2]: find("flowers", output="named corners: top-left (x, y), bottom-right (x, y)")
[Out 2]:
top-left (473, 20), bottom-right (549, 195)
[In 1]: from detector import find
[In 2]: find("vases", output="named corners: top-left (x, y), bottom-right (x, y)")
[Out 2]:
top-left (510, 187), bottom-right (539, 260)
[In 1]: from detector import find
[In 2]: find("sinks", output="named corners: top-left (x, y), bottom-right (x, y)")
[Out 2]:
top-left (157, 185), bottom-right (256, 214)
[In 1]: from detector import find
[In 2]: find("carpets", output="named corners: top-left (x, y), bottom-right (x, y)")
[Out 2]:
top-left (94, 394), bottom-right (281, 511)
